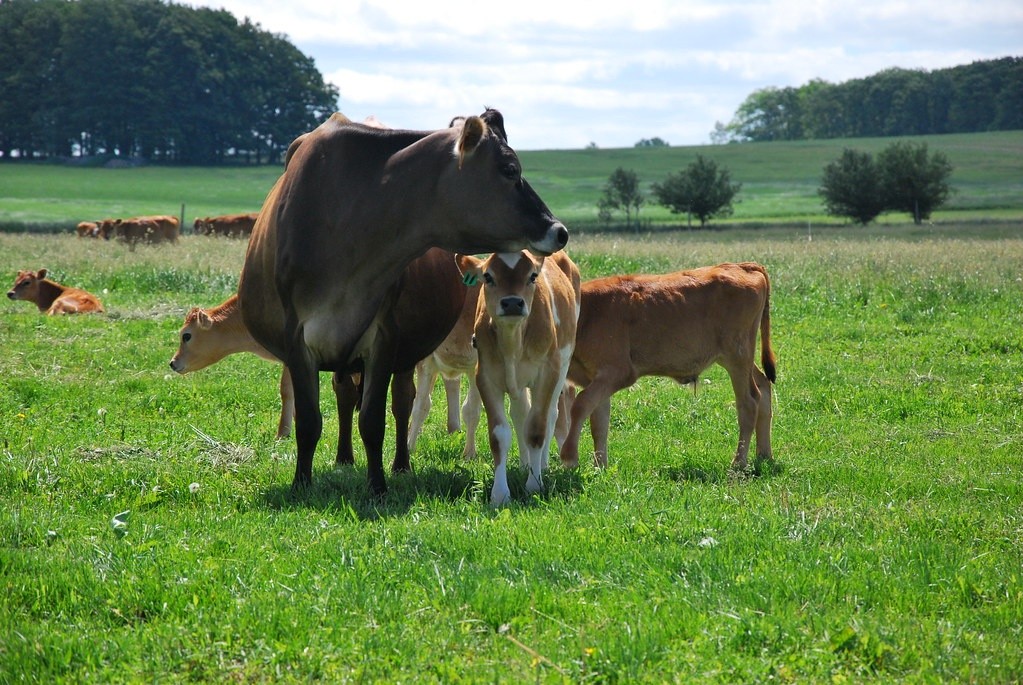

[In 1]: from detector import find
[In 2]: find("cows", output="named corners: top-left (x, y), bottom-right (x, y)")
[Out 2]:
top-left (171, 107), bottom-right (569, 504)
top-left (559, 262), bottom-right (777, 481)
top-left (6, 270), bottom-right (106, 317)
top-left (77, 215), bottom-right (180, 245)
top-left (454, 248), bottom-right (582, 510)
